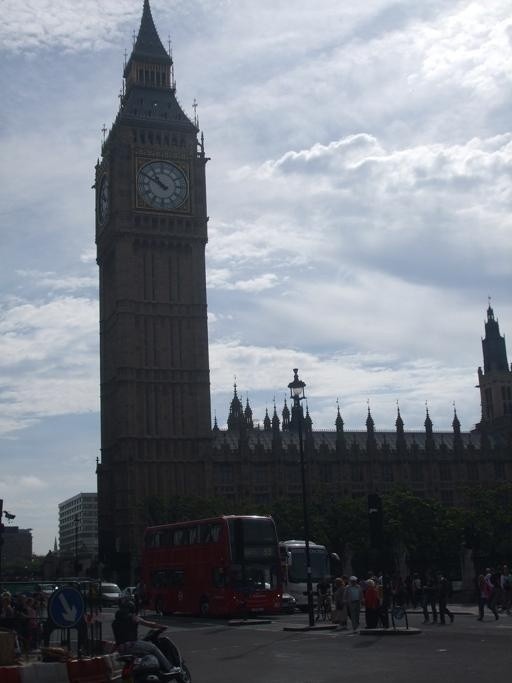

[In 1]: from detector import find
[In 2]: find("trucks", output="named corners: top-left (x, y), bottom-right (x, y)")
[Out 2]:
top-left (442, 547), bottom-right (480, 592)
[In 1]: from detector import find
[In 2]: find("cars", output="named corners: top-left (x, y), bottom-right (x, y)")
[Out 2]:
top-left (39, 581), bottom-right (136, 606)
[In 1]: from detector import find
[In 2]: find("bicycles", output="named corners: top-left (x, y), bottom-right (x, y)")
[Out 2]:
top-left (316, 592), bottom-right (332, 621)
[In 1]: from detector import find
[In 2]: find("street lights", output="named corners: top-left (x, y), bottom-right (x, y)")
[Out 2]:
top-left (288, 369), bottom-right (314, 626)
top-left (73, 516), bottom-right (80, 577)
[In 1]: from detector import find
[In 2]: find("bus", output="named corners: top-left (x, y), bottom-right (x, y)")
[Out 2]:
top-left (141, 514), bottom-right (340, 618)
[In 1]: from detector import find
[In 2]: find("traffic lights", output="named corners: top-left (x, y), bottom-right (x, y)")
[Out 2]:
top-left (461, 526), bottom-right (470, 550)
top-left (367, 493), bottom-right (382, 548)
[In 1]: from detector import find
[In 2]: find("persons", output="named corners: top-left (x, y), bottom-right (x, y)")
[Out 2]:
top-left (110, 598), bottom-right (182, 673)
top-left (0, 587), bottom-right (48, 653)
top-left (312, 564), bottom-right (511, 629)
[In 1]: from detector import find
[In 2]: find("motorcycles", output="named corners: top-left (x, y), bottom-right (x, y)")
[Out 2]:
top-left (115, 627), bottom-right (191, 683)
